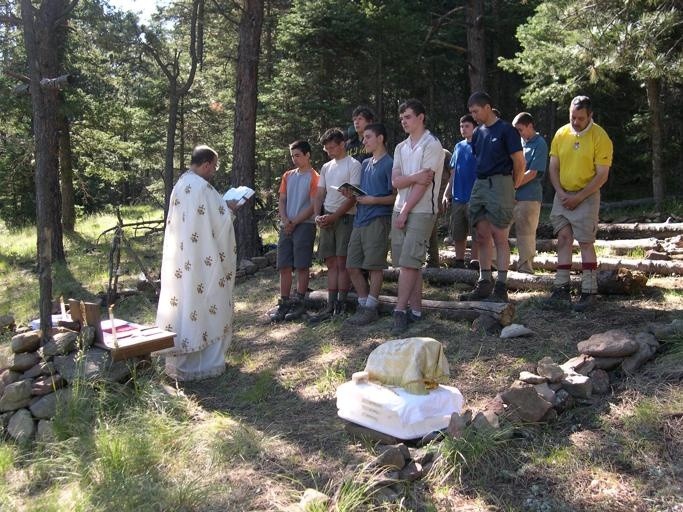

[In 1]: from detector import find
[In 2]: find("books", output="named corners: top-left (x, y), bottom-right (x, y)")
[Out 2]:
top-left (329, 182), bottom-right (367, 198)
top-left (222, 185), bottom-right (255, 205)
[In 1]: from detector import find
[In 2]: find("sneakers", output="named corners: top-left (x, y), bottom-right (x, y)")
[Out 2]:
top-left (459, 277), bottom-right (495, 301)
top-left (550, 283), bottom-right (571, 299)
top-left (452, 259), bottom-right (479, 270)
top-left (488, 280), bottom-right (509, 303)
top-left (271, 296), bottom-right (379, 326)
top-left (573, 294), bottom-right (596, 313)
top-left (390, 308), bottom-right (422, 335)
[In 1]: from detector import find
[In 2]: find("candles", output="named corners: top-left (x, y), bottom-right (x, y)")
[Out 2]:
top-left (59, 295), bottom-right (68, 322)
top-left (108, 303), bottom-right (119, 348)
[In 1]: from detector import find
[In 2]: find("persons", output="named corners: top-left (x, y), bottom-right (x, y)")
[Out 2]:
top-left (441, 113), bottom-right (480, 270)
top-left (270, 139), bottom-right (321, 322)
top-left (156, 144), bottom-right (239, 383)
top-left (343, 104), bottom-right (376, 164)
top-left (336, 122), bottom-right (393, 324)
top-left (313, 128), bottom-right (362, 317)
top-left (390, 97), bottom-right (446, 328)
top-left (426, 147), bottom-right (452, 268)
top-left (512, 112), bottom-right (549, 275)
top-left (492, 107), bottom-right (502, 118)
top-left (459, 90), bottom-right (527, 302)
top-left (548, 95), bottom-right (614, 311)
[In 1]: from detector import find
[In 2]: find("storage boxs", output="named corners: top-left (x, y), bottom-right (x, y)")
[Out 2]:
top-left (337, 376), bottom-right (465, 441)
